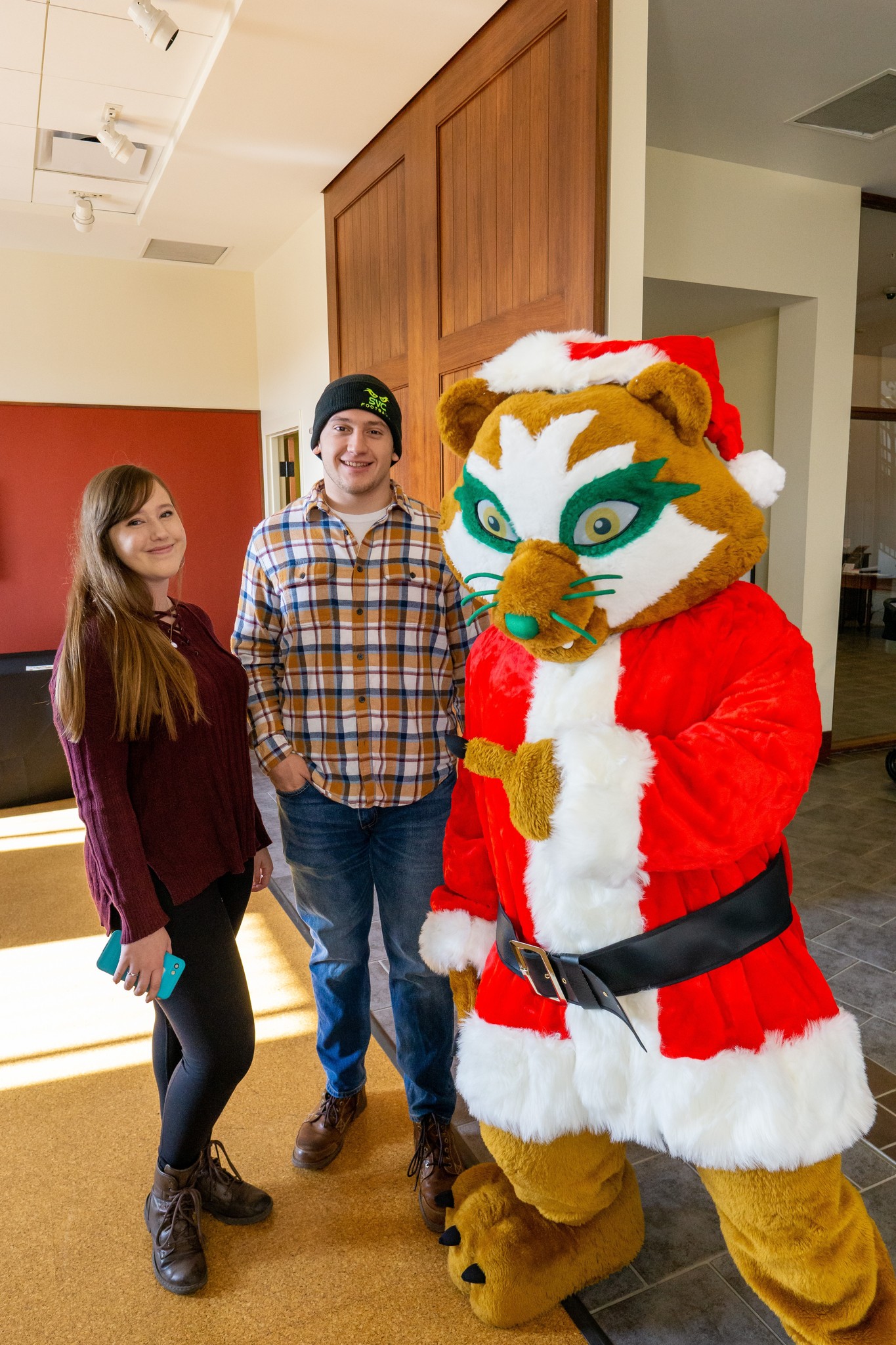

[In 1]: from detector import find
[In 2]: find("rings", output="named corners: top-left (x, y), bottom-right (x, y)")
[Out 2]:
top-left (126, 970), bottom-right (138, 977)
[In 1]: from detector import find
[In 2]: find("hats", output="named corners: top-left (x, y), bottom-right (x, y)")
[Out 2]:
top-left (311, 373), bottom-right (403, 468)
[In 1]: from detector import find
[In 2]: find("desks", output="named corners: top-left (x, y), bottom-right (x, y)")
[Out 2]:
top-left (841, 572), bottom-right (895, 635)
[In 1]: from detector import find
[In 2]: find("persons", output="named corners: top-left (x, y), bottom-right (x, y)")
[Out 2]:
top-left (42, 464), bottom-right (276, 1296)
top-left (231, 372), bottom-right (489, 1238)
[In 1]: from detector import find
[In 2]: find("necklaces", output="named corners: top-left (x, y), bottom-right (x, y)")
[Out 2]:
top-left (155, 597), bottom-right (178, 649)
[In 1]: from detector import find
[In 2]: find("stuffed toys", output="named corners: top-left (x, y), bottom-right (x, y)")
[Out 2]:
top-left (414, 329), bottom-right (895, 1345)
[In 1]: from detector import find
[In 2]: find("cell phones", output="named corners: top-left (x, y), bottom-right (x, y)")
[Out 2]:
top-left (97, 930), bottom-right (186, 1000)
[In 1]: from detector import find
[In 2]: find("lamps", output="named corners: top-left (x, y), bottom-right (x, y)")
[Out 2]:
top-left (127, 0), bottom-right (179, 51)
top-left (68, 189), bottom-right (112, 233)
top-left (95, 102), bottom-right (137, 165)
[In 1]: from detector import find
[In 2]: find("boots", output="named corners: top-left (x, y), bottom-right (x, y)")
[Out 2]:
top-left (407, 1114), bottom-right (465, 1233)
top-left (291, 1081), bottom-right (368, 1168)
top-left (143, 1148), bottom-right (210, 1295)
top-left (195, 1138), bottom-right (273, 1225)
